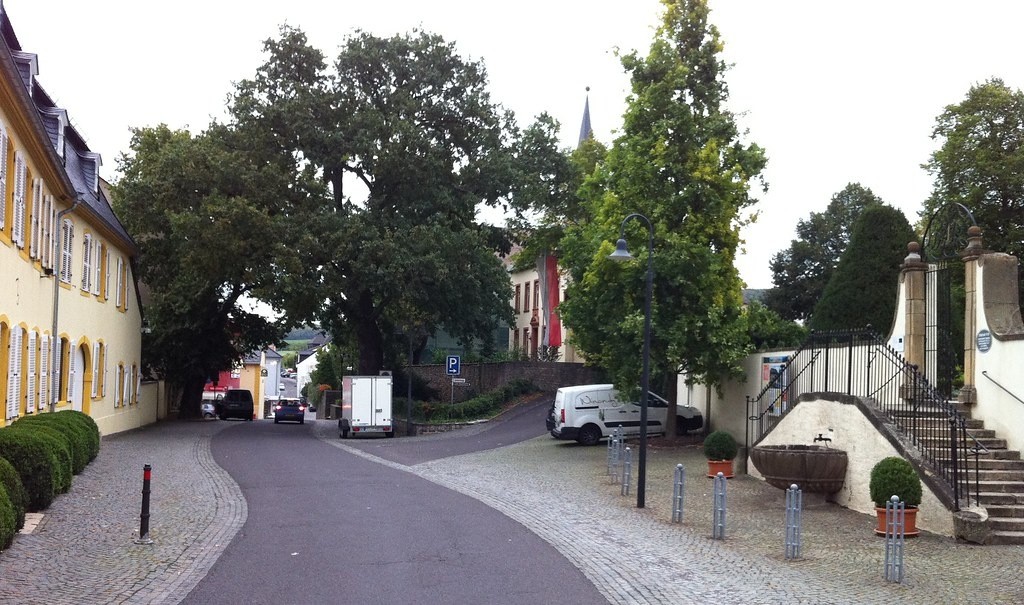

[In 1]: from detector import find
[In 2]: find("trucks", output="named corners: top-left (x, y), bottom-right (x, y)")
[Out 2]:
top-left (200, 385), bottom-right (230, 419)
top-left (338, 376), bottom-right (394, 438)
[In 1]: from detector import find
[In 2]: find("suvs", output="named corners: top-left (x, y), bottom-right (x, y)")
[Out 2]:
top-left (216, 389), bottom-right (254, 420)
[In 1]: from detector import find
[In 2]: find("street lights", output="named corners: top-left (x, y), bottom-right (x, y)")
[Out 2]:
top-left (608, 213), bottom-right (652, 508)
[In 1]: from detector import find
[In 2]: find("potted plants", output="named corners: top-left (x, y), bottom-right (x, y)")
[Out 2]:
top-left (868, 457), bottom-right (922, 535)
top-left (703, 430), bottom-right (739, 478)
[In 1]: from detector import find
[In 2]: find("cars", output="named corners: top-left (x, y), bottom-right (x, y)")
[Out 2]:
top-left (275, 399), bottom-right (305, 424)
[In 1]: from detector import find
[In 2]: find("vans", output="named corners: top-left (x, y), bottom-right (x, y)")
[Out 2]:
top-left (546, 384), bottom-right (704, 445)
